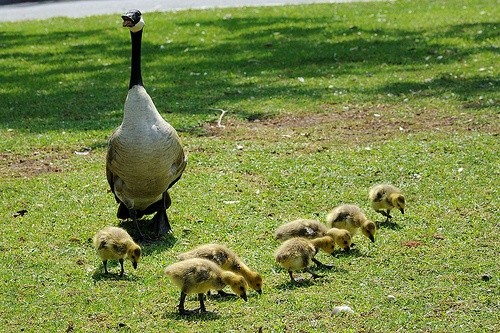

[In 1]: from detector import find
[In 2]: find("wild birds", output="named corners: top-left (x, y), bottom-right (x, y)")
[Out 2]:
top-left (93, 225), bottom-right (142, 279)
top-left (165, 183), bottom-right (405, 318)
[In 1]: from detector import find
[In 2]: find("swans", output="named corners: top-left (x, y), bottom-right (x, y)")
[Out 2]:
top-left (106, 8), bottom-right (188, 247)
